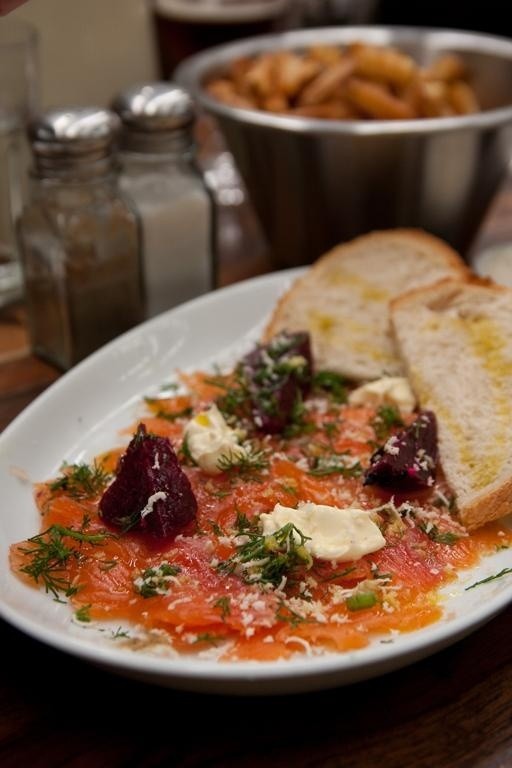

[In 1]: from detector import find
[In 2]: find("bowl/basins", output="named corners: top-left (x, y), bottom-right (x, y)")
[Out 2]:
top-left (170, 24), bottom-right (511, 272)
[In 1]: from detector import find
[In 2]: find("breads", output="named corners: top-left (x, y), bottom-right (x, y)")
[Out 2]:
top-left (260, 226), bottom-right (512, 533)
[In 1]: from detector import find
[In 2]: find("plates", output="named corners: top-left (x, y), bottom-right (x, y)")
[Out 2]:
top-left (0, 262), bottom-right (512, 698)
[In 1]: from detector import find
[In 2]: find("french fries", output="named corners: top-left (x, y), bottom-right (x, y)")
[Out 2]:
top-left (204, 42), bottom-right (478, 124)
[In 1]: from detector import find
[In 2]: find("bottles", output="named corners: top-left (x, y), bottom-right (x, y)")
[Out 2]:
top-left (115, 82), bottom-right (219, 319)
top-left (11, 103), bottom-right (147, 372)
